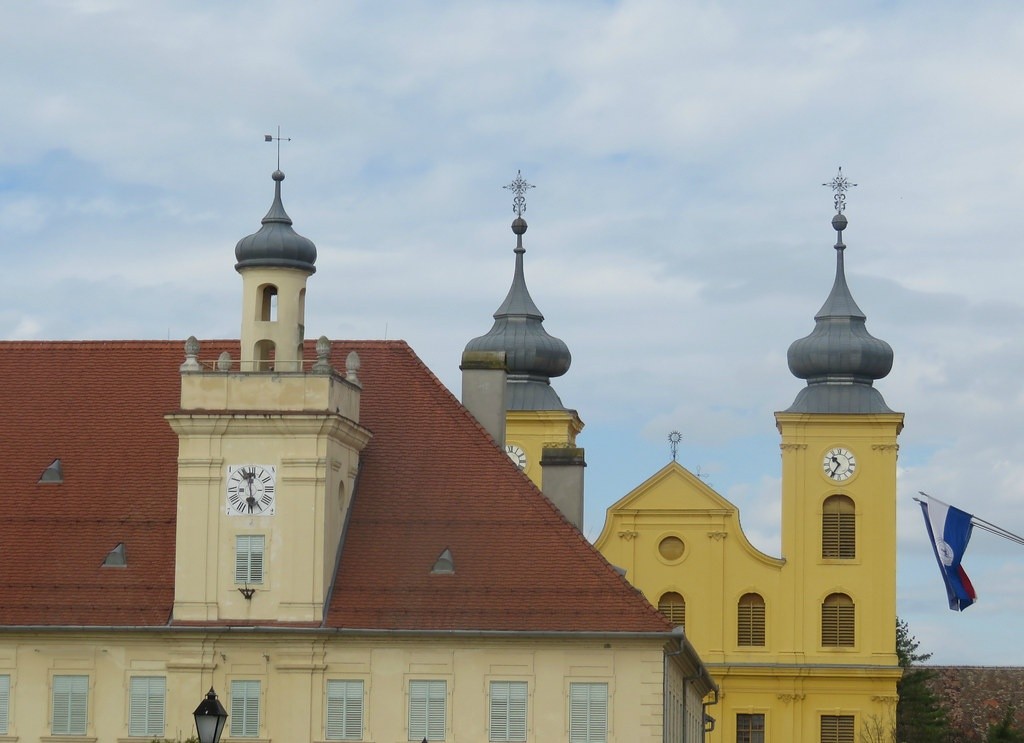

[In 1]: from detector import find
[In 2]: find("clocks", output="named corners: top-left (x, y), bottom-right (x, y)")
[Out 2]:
top-left (504, 446), bottom-right (527, 471)
top-left (817, 442), bottom-right (860, 484)
top-left (227, 464), bottom-right (275, 516)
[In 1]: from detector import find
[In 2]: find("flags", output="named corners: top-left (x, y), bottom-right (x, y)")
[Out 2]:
top-left (918, 495), bottom-right (978, 612)
